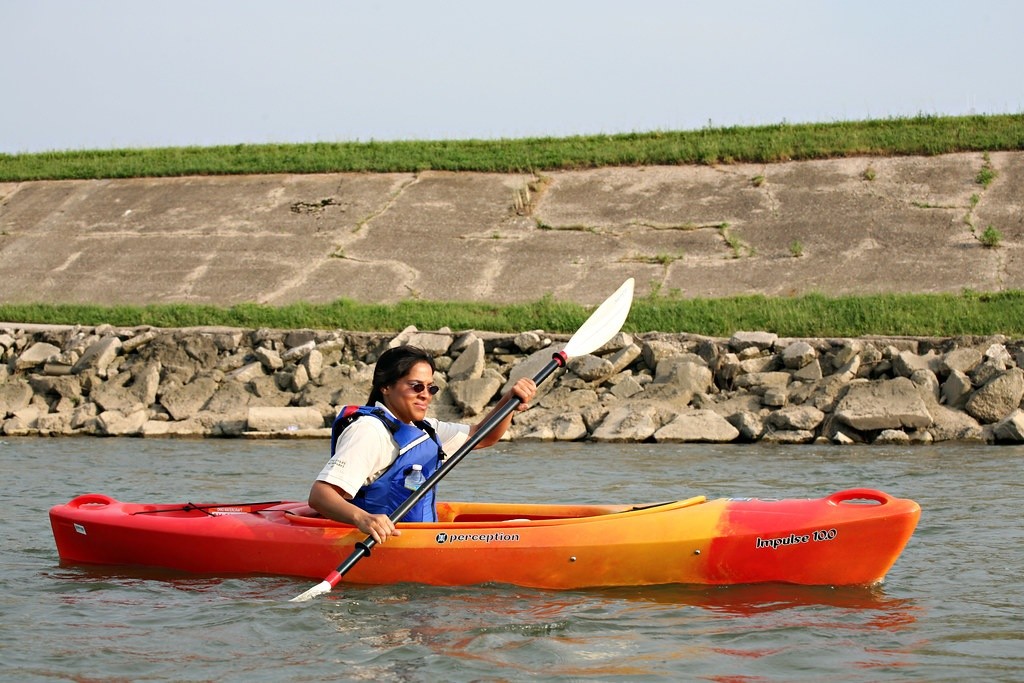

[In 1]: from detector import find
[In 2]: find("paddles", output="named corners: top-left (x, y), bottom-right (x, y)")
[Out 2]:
top-left (285, 276), bottom-right (638, 606)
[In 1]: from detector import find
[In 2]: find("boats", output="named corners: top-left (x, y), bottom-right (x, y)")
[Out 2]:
top-left (49, 490), bottom-right (917, 585)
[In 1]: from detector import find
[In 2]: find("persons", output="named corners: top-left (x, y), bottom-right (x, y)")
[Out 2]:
top-left (310, 345), bottom-right (536, 544)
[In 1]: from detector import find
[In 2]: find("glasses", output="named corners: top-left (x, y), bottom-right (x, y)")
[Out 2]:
top-left (396, 380), bottom-right (441, 395)
